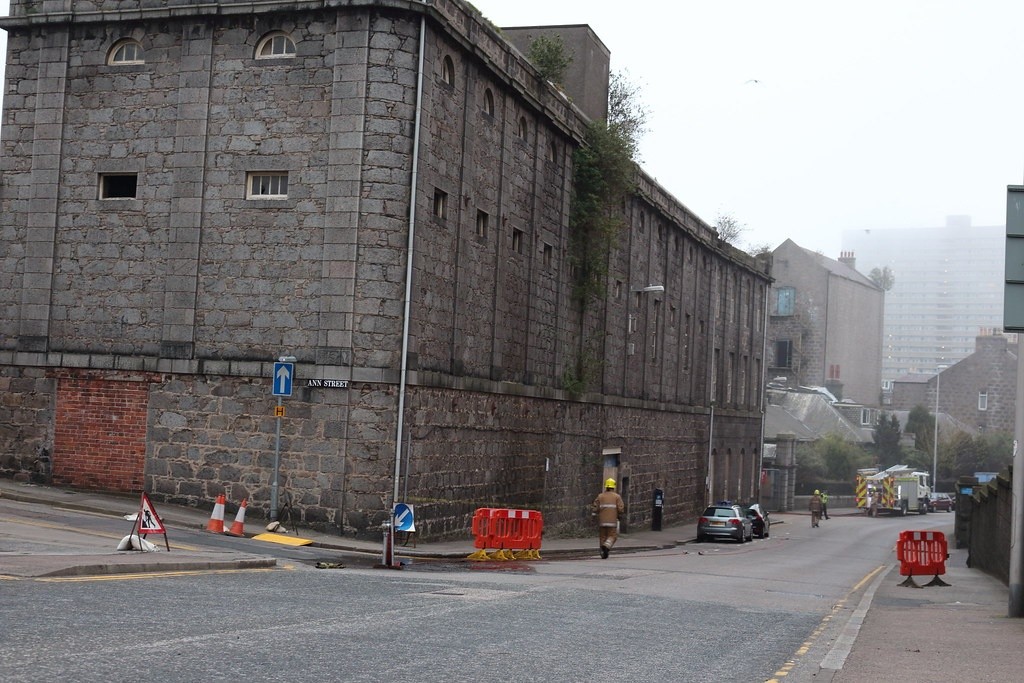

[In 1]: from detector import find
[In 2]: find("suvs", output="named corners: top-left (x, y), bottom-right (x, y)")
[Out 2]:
top-left (927, 492), bottom-right (953, 513)
top-left (696, 503), bottom-right (754, 542)
top-left (740, 503), bottom-right (770, 537)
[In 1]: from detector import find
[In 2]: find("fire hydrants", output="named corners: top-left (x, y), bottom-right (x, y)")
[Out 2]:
top-left (379, 520), bottom-right (393, 568)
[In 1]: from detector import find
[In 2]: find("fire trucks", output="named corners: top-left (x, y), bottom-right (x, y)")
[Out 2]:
top-left (856, 463), bottom-right (932, 516)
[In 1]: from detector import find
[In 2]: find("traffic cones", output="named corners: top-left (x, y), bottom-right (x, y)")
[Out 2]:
top-left (228, 500), bottom-right (250, 536)
top-left (206, 494), bottom-right (226, 533)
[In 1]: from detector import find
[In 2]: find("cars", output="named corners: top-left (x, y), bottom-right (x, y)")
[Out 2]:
top-left (949, 494), bottom-right (956, 507)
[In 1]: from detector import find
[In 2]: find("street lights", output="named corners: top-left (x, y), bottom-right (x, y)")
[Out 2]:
top-left (933, 365), bottom-right (948, 513)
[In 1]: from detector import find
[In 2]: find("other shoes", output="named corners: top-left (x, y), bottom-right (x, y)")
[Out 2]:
top-left (826, 517), bottom-right (831, 520)
top-left (600, 545), bottom-right (609, 559)
top-left (819, 518), bottom-right (824, 520)
top-left (815, 524), bottom-right (819, 527)
top-left (812, 525), bottom-right (815, 527)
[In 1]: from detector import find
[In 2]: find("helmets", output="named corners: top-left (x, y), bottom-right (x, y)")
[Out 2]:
top-left (814, 490), bottom-right (820, 495)
top-left (605, 477), bottom-right (616, 488)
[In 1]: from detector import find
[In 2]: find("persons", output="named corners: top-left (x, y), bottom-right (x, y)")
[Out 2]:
top-left (592, 478), bottom-right (624, 559)
top-left (820, 489), bottom-right (831, 520)
top-left (809, 490), bottom-right (823, 528)
